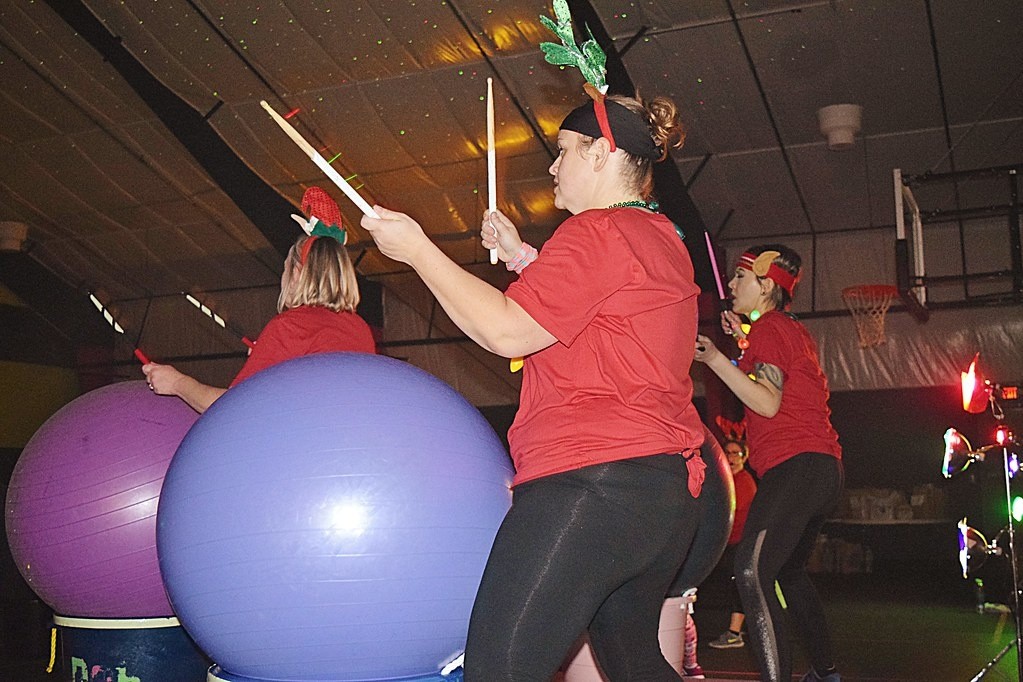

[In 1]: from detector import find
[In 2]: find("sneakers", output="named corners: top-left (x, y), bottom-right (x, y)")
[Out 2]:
top-left (799, 665), bottom-right (842, 682)
top-left (709, 630), bottom-right (746, 648)
top-left (680, 663), bottom-right (705, 679)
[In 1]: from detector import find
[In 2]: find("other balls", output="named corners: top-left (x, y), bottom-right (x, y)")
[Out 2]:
top-left (4, 377), bottom-right (205, 621)
top-left (154, 353), bottom-right (518, 681)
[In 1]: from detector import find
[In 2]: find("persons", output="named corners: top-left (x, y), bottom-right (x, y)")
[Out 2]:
top-left (564, 421), bottom-right (737, 682)
top-left (694, 243), bottom-right (848, 682)
top-left (708, 439), bottom-right (758, 649)
top-left (141, 231), bottom-right (377, 414)
top-left (359, 92), bottom-right (710, 682)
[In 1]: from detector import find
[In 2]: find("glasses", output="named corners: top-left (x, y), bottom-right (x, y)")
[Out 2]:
top-left (724, 448), bottom-right (745, 458)
